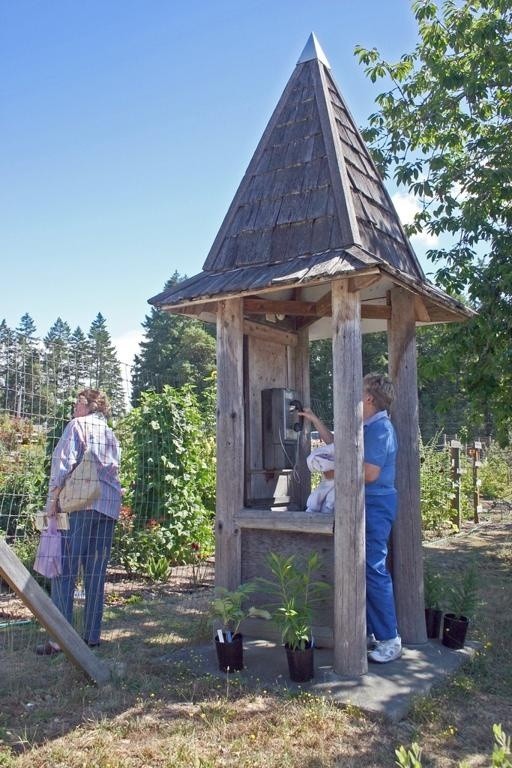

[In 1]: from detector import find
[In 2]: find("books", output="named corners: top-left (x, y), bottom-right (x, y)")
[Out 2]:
top-left (34, 511), bottom-right (71, 531)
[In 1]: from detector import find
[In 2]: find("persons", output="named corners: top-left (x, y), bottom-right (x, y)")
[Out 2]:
top-left (292, 367), bottom-right (404, 666)
top-left (33, 384), bottom-right (123, 657)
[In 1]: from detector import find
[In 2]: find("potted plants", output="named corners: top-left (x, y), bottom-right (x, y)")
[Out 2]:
top-left (423, 555), bottom-right (479, 650)
top-left (213, 552), bottom-right (332, 682)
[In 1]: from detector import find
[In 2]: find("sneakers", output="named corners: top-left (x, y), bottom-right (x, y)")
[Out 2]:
top-left (367, 633), bottom-right (405, 665)
top-left (35, 641), bottom-right (64, 655)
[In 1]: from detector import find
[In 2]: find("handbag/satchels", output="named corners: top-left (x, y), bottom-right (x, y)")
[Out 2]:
top-left (32, 515), bottom-right (64, 579)
top-left (58, 418), bottom-right (100, 513)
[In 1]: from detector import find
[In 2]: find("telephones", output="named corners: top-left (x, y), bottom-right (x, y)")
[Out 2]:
top-left (262, 389), bottom-right (304, 469)
